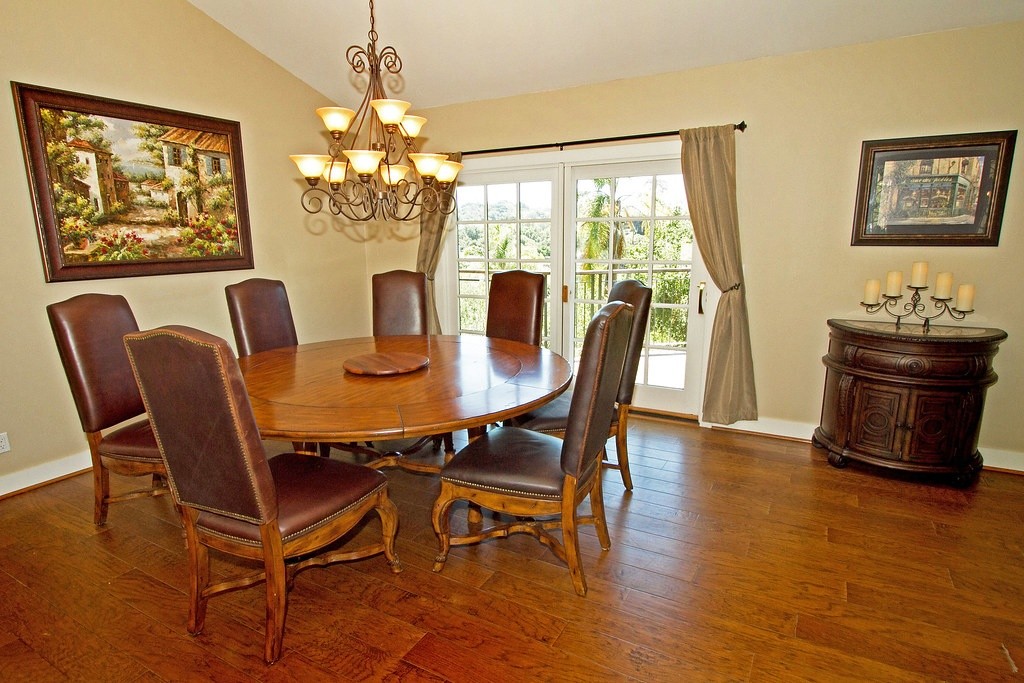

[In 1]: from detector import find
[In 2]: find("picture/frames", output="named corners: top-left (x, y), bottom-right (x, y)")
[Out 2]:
top-left (850, 129), bottom-right (1019, 247)
top-left (8, 80), bottom-right (255, 283)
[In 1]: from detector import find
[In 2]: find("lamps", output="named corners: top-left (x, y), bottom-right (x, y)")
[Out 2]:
top-left (288, 0), bottom-right (463, 221)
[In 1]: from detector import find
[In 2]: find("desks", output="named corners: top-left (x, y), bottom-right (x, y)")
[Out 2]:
top-left (235, 335), bottom-right (572, 525)
top-left (810, 318), bottom-right (1009, 492)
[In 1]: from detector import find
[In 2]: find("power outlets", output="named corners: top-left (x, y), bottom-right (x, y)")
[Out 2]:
top-left (0, 432), bottom-right (11, 454)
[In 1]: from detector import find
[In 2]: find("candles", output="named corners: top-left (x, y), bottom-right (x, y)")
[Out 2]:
top-left (910, 262), bottom-right (929, 288)
top-left (863, 279), bottom-right (880, 305)
top-left (886, 271), bottom-right (903, 296)
top-left (955, 284), bottom-right (975, 311)
top-left (933, 271), bottom-right (953, 299)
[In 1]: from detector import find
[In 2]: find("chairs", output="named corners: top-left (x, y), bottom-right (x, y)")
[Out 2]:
top-left (123, 325), bottom-right (403, 668)
top-left (431, 300), bottom-right (635, 595)
top-left (503, 277), bottom-right (654, 490)
top-left (226, 278), bottom-right (332, 461)
top-left (47, 292), bottom-right (189, 550)
top-left (359, 269), bottom-right (456, 460)
top-left (486, 270), bottom-right (549, 426)
